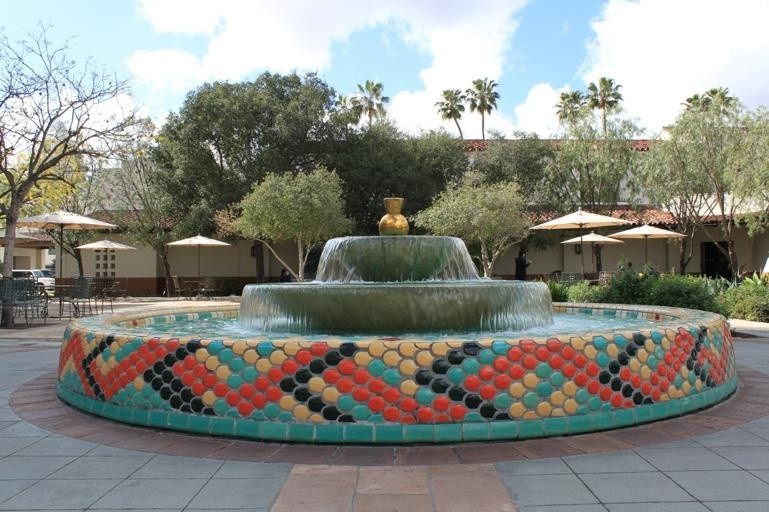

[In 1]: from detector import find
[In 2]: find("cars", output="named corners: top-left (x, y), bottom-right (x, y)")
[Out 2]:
top-left (12, 270), bottom-right (55, 291)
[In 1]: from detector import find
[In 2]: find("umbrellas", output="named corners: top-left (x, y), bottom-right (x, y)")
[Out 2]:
top-left (607, 223), bottom-right (689, 263)
top-left (0, 227), bottom-right (42, 248)
top-left (528, 206), bottom-right (631, 279)
top-left (165, 232), bottom-right (234, 277)
top-left (14, 208), bottom-right (124, 286)
top-left (73, 238), bottom-right (140, 277)
top-left (558, 230), bottom-right (626, 273)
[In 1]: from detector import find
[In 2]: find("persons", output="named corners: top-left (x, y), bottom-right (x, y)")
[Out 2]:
top-left (514, 251), bottom-right (532, 279)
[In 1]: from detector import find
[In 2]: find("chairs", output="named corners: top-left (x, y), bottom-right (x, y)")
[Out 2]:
top-left (171, 276), bottom-right (217, 301)
top-left (0, 275), bottom-right (114, 330)
top-left (556, 272), bottom-right (615, 287)
